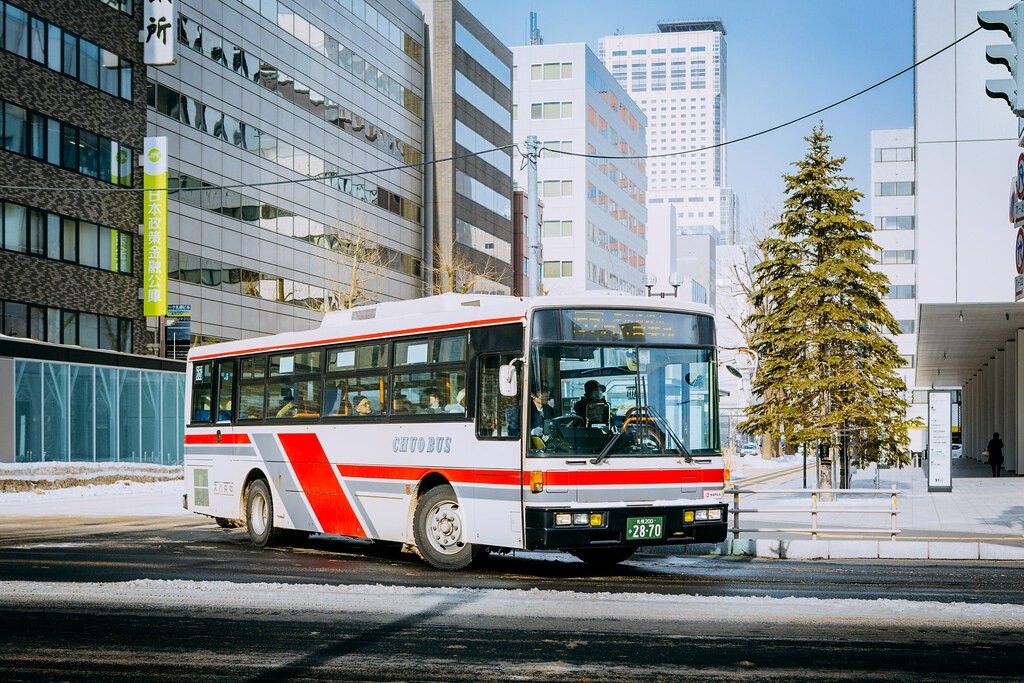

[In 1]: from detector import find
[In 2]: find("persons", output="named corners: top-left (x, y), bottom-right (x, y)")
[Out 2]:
top-left (504, 379), bottom-right (562, 454)
top-left (239, 374), bottom-right (476, 425)
top-left (573, 380), bottom-right (618, 431)
top-left (986, 432), bottom-right (1005, 477)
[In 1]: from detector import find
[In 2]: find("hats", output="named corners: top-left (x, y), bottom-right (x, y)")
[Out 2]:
top-left (584, 380), bottom-right (606, 392)
top-left (531, 380), bottom-right (553, 393)
top-left (352, 395), bottom-right (368, 405)
top-left (392, 389), bottom-right (406, 399)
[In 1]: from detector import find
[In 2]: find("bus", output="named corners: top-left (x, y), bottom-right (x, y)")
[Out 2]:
top-left (180, 290), bottom-right (742, 572)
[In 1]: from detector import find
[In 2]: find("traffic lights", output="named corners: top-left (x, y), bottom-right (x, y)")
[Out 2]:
top-left (976, 2), bottom-right (1024, 120)
